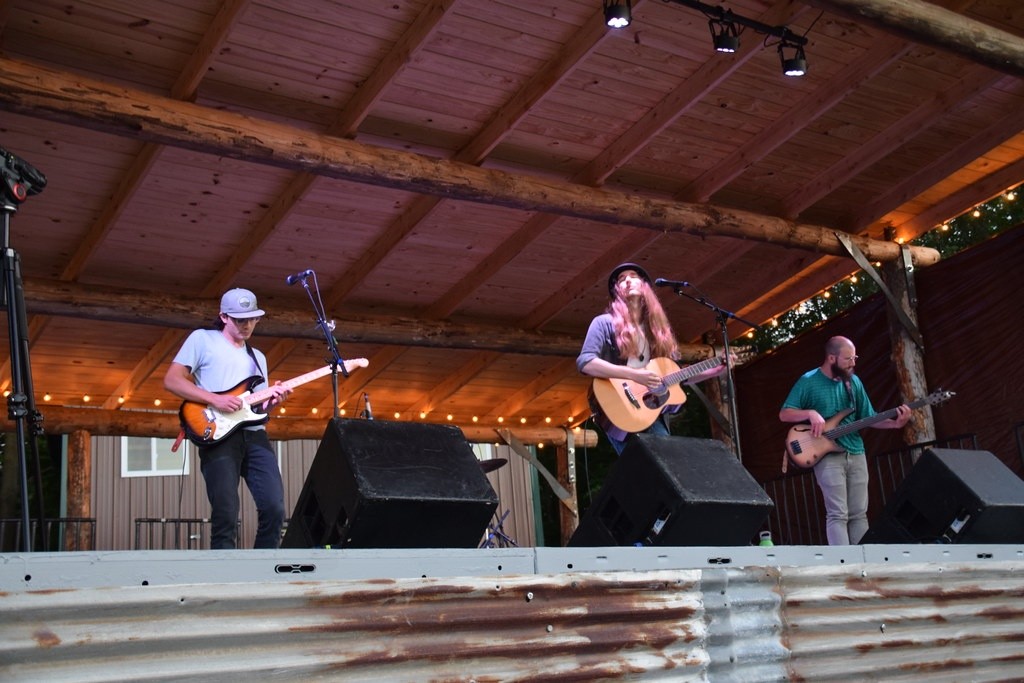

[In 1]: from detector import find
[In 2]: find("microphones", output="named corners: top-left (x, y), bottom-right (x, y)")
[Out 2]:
top-left (364, 393), bottom-right (373, 420)
top-left (286, 269), bottom-right (313, 286)
top-left (655, 278), bottom-right (691, 287)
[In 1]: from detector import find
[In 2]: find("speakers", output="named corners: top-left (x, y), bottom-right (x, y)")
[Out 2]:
top-left (563, 434), bottom-right (774, 548)
top-left (858, 447), bottom-right (1024, 545)
top-left (279, 417), bottom-right (500, 548)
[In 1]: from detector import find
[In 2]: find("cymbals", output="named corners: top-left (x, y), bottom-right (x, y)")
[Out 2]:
top-left (479, 458), bottom-right (507, 473)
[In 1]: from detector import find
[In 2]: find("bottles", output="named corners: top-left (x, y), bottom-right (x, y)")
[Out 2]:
top-left (759, 531), bottom-right (775, 546)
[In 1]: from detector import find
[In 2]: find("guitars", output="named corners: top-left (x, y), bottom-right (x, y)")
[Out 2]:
top-left (179, 357), bottom-right (370, 448)
top-left (786, 388), bottom-right (957, 472)
top-left (587, 344), bottom-right (758, 440)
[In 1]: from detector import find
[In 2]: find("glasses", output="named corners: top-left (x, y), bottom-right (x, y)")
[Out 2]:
top-left (224, 314), bottom-right (262, 325)
top-left (830, 353), bottom-right (858, 361)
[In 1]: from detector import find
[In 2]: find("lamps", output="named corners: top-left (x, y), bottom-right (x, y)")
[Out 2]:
top-left (776, 42), bottom-right (807, 76)
top-left (709, 18), bottom-right (738, 53)
top-left (603, 0), bottom-right (632, 28)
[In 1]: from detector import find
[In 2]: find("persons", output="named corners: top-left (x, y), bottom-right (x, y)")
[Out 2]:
top-left (779, 335), bottom-right (912, 545)
top-left (575, 263), bottom-right (738, 456)
top-left (164, 288), bottom-right (294, 550)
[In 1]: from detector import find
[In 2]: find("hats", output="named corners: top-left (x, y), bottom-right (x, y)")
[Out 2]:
top-left (220, 287), bottom-right (265, 319)
top-left (608, 263), bottom-right (653, 302)
